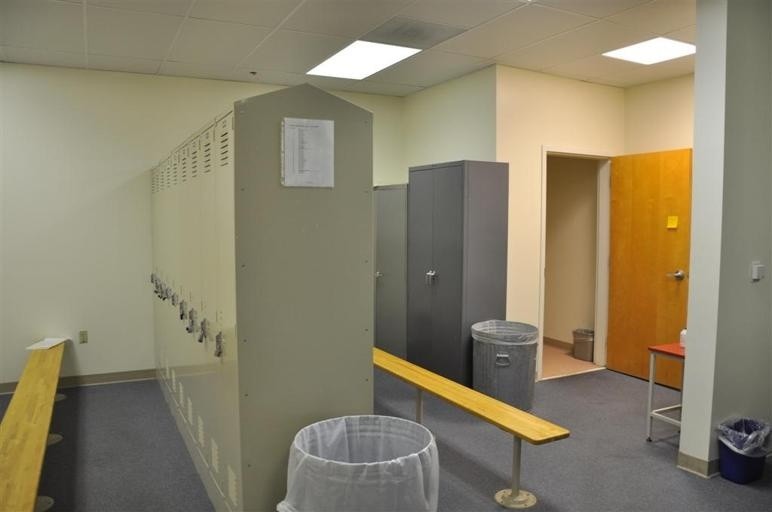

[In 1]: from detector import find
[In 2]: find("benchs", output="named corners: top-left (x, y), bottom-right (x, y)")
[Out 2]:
top-left (373, 347), bottom-right (573, 509)
top-left (0, 337), bottom-right (66, 512)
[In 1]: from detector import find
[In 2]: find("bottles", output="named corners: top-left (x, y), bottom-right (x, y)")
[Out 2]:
top-left (680, 328), bottom-right (687, 348)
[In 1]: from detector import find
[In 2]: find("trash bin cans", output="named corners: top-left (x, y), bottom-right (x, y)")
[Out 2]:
top-left (572, 328), bottom-right (595, 362)
top-left (718, 414), bottom-right (772, 485)
top-left (471, 320), bottom-right (540, 411)
top-left (285, 414), bottom-right (441, 512)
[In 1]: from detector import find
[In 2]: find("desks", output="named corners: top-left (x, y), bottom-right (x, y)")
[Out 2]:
top-left (647, 342), bottom-right (686, 443)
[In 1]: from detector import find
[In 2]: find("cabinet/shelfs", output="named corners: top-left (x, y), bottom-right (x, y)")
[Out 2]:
top-left (149, 83), bottom-right (375, 512)
top-left (375, 159), bottom-right (510, 385)
